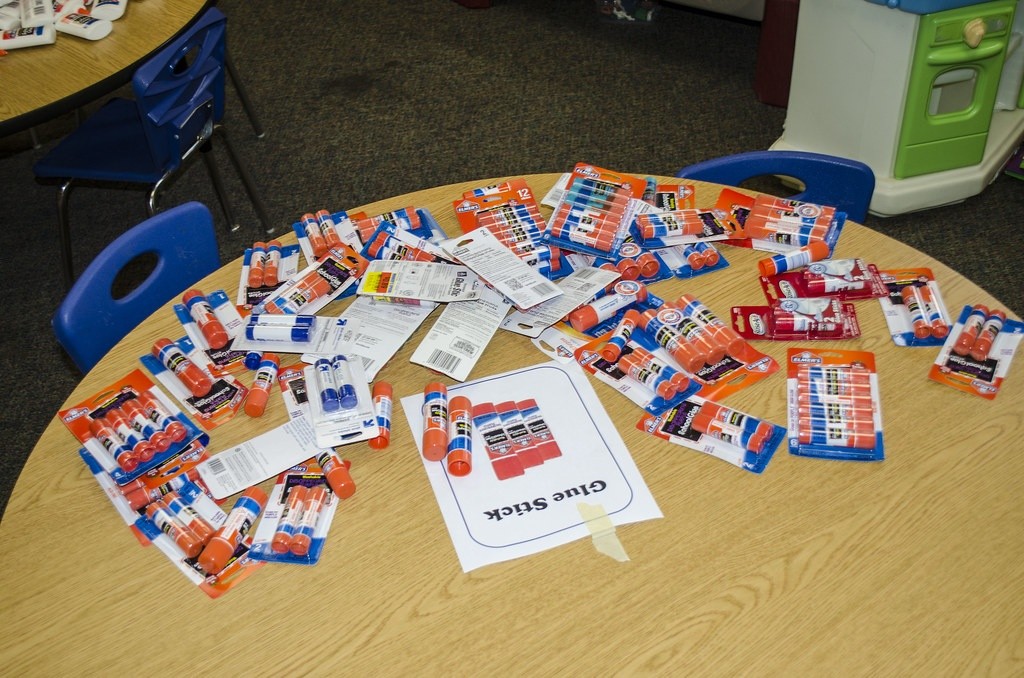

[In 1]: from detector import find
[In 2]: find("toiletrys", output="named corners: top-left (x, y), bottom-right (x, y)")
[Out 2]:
top-left (54, 12), bottom-right (114, 41)
top-left (91, 0), bottom-right (128, 21)
top-left (0, 0), bottom-right (92, 28)
top-left (0, 27), bottom-right (56, 51)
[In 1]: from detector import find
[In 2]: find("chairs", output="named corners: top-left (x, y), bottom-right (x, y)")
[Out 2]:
top-left (676, 151), bottom-right (875, 226)
top-left (51, 200), bottom-right (220, 375)
top-left (31, 7), bottom-right (275, 294)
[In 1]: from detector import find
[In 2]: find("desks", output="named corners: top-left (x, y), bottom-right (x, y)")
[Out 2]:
top-left (0, 175), bottom-right (1024, 678)
top-left (0, 0), bottom-right (221, 138)
